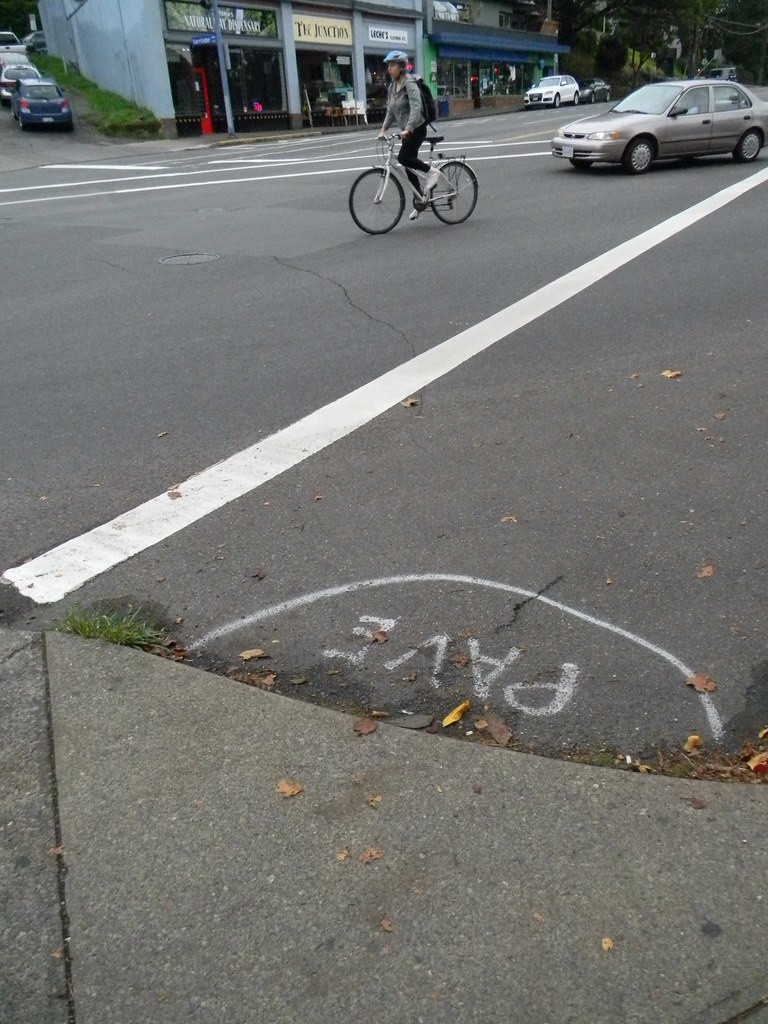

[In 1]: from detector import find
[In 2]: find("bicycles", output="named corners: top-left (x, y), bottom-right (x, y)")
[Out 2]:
top-left (348, 131), bottom-right (479, 235)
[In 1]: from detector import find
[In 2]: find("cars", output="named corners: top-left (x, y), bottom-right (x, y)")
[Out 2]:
top-left (577, 78), bottom-right (613, 104)
top-left (0, 29), bottom-right (75, 133)
top-left (708, 67), bottom-right (738, 83)
top-left (549, 77), bottom-right (768, 176)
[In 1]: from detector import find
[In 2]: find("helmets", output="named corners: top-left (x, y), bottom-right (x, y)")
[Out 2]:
top-left (383, 51), bottom-right (408, 65)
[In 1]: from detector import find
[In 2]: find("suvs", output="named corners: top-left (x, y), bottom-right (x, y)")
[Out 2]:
top-left (523, 74), bottom-right (580, 110)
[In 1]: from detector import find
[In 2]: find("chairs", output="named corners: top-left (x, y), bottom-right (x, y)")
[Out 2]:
top-left (321, 107), bottom-right (355, 128)
top-left (686, 94), bottom-right (708, 114)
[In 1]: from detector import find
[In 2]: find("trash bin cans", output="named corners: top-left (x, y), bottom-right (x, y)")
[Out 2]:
top-left (439, 98), bottom-right (449, 118)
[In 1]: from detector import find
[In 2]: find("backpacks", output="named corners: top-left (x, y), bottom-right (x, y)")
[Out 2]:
top-left (404, 79), bottom-right (436, 124)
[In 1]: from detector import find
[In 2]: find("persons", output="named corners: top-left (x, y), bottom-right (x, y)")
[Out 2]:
top-left (378, 51), bottom-right (440, 220)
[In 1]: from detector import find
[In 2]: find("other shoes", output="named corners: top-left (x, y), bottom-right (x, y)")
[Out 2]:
top-left (408, 209), bottom-right (418, 220)
top-left (426, 165), bottom-right (441, 189)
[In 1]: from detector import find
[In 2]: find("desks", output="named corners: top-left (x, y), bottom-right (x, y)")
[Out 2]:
top-left (312, 107), bottom-right (360, 128)
